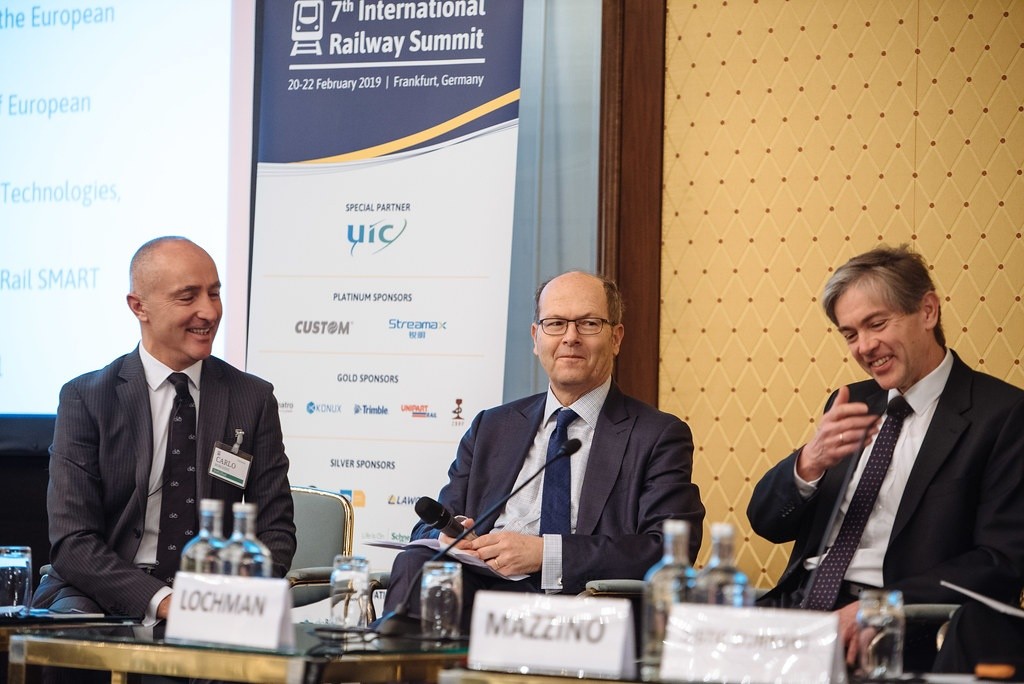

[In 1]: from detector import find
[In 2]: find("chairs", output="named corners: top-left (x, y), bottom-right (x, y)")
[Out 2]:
top-left (281, 480), bottom-right (356, 622)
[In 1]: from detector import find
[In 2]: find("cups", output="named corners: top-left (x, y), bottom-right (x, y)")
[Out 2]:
top-left (420, 560), bottom-right (463, 651)
top-left (328, 554), bottom-right (370, 644)
top-left (0, 546), bottom-right (33, 625)
top-left (856, 589), bottom-right (905, 681)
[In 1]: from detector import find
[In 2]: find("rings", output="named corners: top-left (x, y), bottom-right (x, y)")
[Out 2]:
top-left (494, 559), bottom-right (500, 569)
top-left (840, 433), bottom-right (843, 443)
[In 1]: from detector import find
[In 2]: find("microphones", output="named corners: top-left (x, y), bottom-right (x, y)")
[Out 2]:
top-left (374, 439), bottom-right (583, 634)
top-left (414, 494), bottom-right (479, 540)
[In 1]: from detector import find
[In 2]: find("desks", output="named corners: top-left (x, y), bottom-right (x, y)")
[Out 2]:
top-left (7, 621), bottom-right (640, 684)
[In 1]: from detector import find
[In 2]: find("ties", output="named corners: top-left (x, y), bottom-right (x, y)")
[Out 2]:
top-left (795, 396), bottom-right (914, 611)
top-left (152, 371), bottom-right (199, 580)
top-left (536, 411), bottom-right (578, 538)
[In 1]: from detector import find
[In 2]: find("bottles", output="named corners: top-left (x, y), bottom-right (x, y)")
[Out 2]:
top-left (641, 517), bottom-right (697, 683)
top-left (688, 522), bottom-right (756, 607)
top-left (212, 502), bottom-right (274, 578)
top-left (180, 498), bottom-right (227, 576)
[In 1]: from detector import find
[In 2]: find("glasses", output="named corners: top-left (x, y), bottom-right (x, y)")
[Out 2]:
top-left (537, 318), bottom-right (617, 336)
top-left (303, 626), bottom-right (383, 644)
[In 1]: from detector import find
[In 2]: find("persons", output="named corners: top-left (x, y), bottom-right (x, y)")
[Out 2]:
top-left (32, 235), bottom-right (297, 684)
top-left (746, 242), bottom-right (1024, 684)
top-left (383, 271), bottom-right (706, 632)
